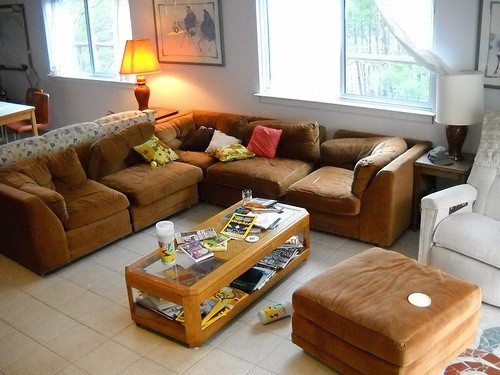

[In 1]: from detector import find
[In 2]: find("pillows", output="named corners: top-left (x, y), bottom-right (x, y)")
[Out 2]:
top-left (180, 128), bottom-right (212, 151)
top-left (132, 135), bottom-right (179, 167)
top-left (247, 123), bottom-right (281, 158)
top-left (214, 144), bottom-right (255, 164)
top-left (206, 130), bottom-right (239, 153)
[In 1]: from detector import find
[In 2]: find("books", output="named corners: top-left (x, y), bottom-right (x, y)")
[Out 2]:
top-left (200, 240), bottom-right (227, 251)
top-left (246, 210), bottom-right (279, 230)
top-left (249, 205), bottom-right (283, 212)
top-left (203, 234), bottom-right (232, 246)
top-left (220, 213), bottom-right (256, 239)
top-left (243, 197), bottom-right (278, 209)
top-left (231, 230), bottom-right (305, 293)
top-left (180, 240), bottom-right (214, 263)
top-left (138, 265), bottom-right (249, 331)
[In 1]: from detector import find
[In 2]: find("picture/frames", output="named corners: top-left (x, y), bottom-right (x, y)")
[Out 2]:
top-left (151, 0), bottom-right (226, 67)
top-left (476, 0), bottom-right (499, 89)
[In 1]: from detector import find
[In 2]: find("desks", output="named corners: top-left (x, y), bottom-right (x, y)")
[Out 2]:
top-left (1, 101), bottom-right (38, 135)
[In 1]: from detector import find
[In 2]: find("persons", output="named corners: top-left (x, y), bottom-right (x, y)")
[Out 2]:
top-left (199, 9), bottom-right (215, 41)
top-left (183, 5), bottom-right (197, 34)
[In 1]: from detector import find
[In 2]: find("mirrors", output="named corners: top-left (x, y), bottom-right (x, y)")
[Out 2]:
top-left (408, 293), bottom-right (432, 308)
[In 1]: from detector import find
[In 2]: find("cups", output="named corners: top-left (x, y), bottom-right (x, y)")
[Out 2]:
top-left (256, 300), bottom-right (293, 325)
top-left (155, 221), bottom-right (175, 263)
top-left (242, 189), bottom-right (253, 204)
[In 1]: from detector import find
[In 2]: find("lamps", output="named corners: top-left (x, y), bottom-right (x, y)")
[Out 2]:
top-left (119, 38), bottom-right (161, 110)
top-left (434, 70), bottom-right (485, 158)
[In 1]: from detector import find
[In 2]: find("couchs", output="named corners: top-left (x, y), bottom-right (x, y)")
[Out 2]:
top-left (0, 110), bottom-right (432, 278)
top-left (289, 245), bottom-right (483, 371)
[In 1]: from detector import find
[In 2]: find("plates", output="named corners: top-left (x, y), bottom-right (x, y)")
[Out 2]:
top-left (245, 236), bottom-right (259, 242)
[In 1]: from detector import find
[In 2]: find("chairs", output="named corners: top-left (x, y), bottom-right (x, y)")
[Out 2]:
top-left (418, 112), bottom-right (499, 306)
top-left (6, 93), bottom-right (50, 138)
top-left (25, 87), bottom-right (42, 104)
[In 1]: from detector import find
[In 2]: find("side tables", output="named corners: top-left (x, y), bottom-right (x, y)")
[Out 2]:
top-left (411, 146), bottom-right (476, 232)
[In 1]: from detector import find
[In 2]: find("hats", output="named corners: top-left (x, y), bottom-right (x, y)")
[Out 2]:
top-left (427, 145), bottom-right (454, 166)
